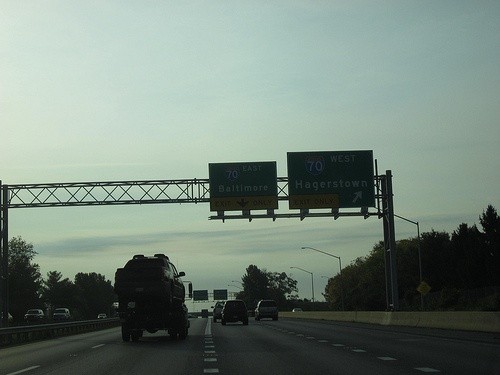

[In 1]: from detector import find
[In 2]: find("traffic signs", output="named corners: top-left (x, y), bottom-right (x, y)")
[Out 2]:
top-left (208, 161), bottom-right (279, 212)
top-left (287, 149), bottom-right (376, 210)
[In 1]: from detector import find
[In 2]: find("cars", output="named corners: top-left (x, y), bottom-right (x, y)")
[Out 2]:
top-left (1, 312), bottom-right (13, 322)
top-left (98, 313), bottom-right (107, 319)
top-left (292, 308), bottom-right (303, 312)
top-left (247, 306), bottom-right (255, 317)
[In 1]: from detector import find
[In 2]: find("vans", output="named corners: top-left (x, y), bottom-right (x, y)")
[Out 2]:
top-left (52, 307), bottom-right (71, 321)
top-left (23, 309), bottom-right (45, 322)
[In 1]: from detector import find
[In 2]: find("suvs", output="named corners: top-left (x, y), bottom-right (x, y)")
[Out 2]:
top-left (255, 299), bottom-right (278, 320)
top-left (210, 301), bottom-right (225, 323)
top-left (220, 300), bottom-right (249, 325)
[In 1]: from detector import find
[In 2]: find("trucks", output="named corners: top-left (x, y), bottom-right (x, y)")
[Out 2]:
top-left (114, 252), bottom-right (194, 343)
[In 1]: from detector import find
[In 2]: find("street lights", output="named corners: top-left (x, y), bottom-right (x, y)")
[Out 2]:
top-left (290, 266), bottom-right (315, 311)
top-left (301, 247), bottom-right (345, 311)
